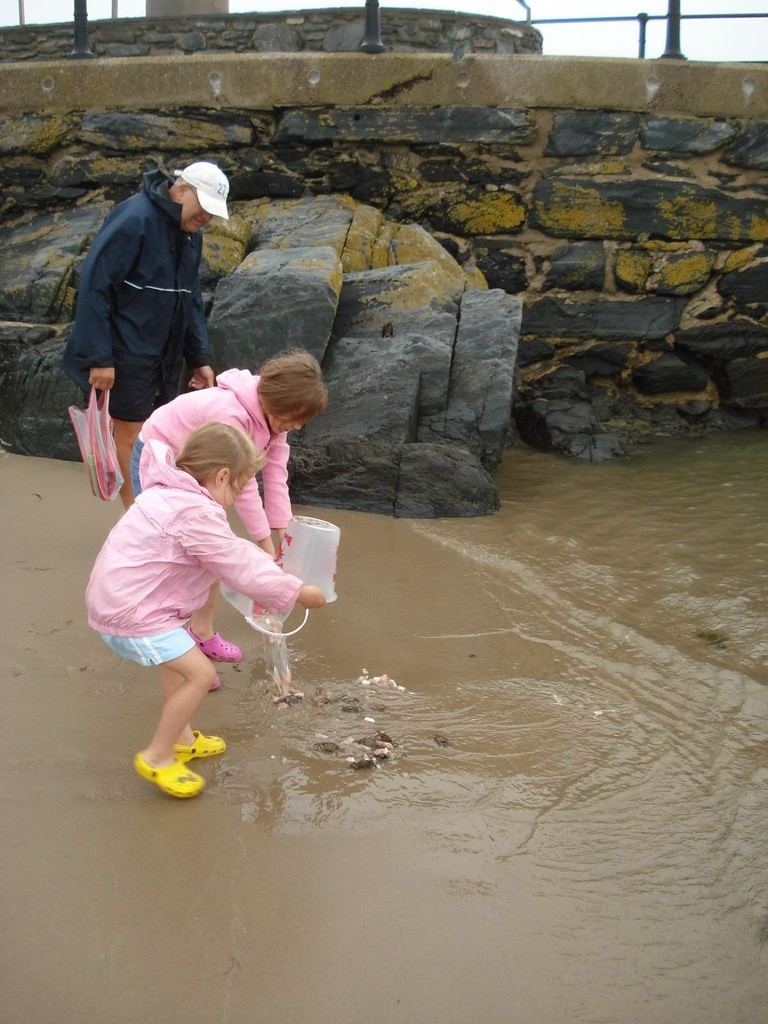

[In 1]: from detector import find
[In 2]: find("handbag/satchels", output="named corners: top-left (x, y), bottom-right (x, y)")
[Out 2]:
top-left (68, 381), bottom-right (123, 503)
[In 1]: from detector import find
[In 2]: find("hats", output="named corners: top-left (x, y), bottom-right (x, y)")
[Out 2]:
top-left (173, 160), bottom-right (231, 222)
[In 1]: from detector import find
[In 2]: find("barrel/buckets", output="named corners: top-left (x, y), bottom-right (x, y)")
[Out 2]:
top-left (219, 576), bottom-right (309, 636)
top-left (271, 515), bottom-right (341, 603)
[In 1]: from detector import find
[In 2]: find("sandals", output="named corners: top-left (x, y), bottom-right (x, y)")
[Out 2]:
top-left (188, 626), bottom-right (245, 663)
top-left (133, 749), bottom-right (207, 799)
top-left (212, 681), bottom-right (220, 691)
top-left (173, 730), bottom-right (227, 763)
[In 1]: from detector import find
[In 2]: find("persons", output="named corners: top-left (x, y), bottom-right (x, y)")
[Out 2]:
top-left (130, 352), bottom-right (329, 687)
top-left (86, 419), bottom-right (326, 799)
top-left (61, 161), bottom-right (231, 511)
